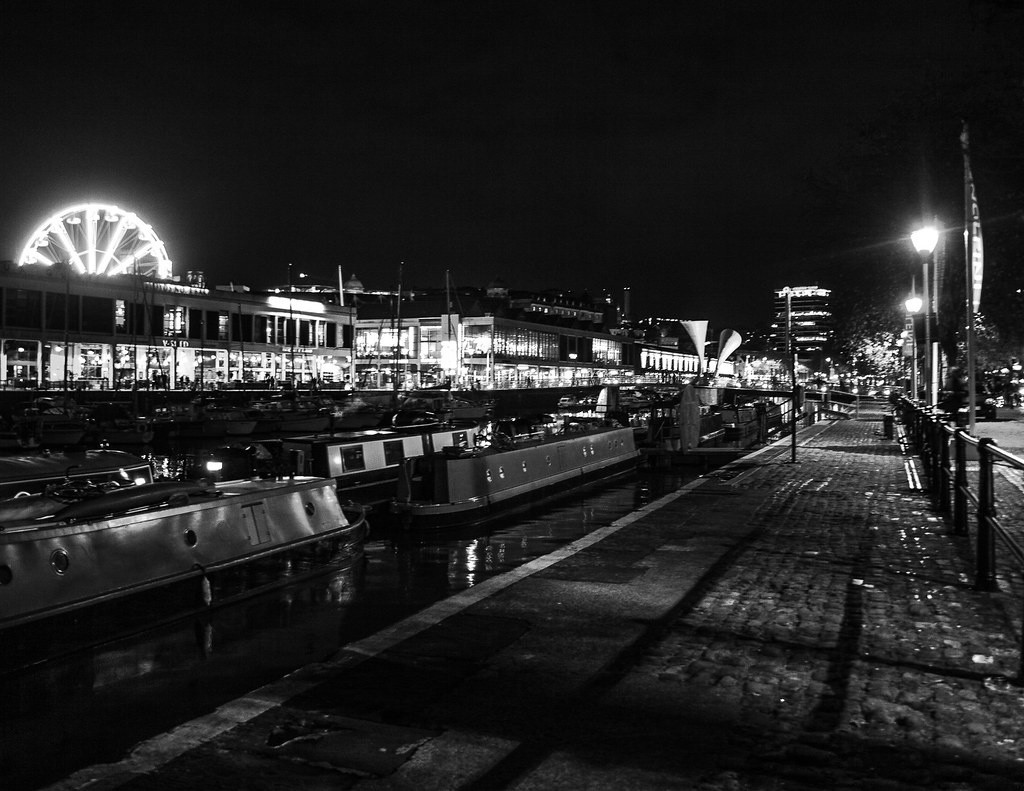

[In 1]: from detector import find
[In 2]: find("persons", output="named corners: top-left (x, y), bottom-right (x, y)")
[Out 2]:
top-left (269, 376), bottom-right (275, 391)
top-left (180, 375), bottom-right (190, 390)
top-left (310, 378), bottom-right (324, 390)
top-left (456, 371), bottom-right (599, 391)
top-left (937, 368), bottom-right (970, 435)
top-left (840, 379), bottom-right (854, 393)
top-left (816, 374), bottom-right (823, 390)
top-left (771, 375), bottom-right (778, 391)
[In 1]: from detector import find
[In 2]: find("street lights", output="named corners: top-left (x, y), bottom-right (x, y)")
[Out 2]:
top-left (904, 291), bottom-right (921, 404)
top-left (908, 224), bottom-right (942, 417)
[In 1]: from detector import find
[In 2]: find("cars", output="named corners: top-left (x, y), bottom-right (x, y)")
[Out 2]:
top-left (920, 367), bottom-right (998, 421)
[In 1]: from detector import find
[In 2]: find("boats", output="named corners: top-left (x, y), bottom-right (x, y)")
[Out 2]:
top-left (0, 264), bottom-right (788, 695)
